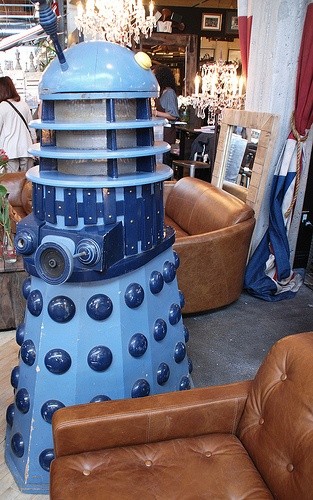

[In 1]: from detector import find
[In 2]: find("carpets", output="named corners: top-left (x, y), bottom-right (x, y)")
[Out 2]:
top-left (183, 285), bottom-right (313, 388)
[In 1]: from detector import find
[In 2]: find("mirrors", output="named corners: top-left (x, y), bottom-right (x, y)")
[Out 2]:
top-left (211, 108), bottom-right (281, 265)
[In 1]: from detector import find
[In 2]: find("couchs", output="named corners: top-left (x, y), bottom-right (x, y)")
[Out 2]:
top-left (163, 177), bottom-right (256, 313)
top-left (0, 172), bottom-right (32, 233)
top-left (50, 333), bottom-right (313, 500)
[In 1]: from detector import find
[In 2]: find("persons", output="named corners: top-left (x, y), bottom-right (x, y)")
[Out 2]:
top-left (0, 76), bottom-right (37, 173)
top-left (151, 65), bottom-right (180, 181)
top-left (189, 133), bottom-right (218, 169)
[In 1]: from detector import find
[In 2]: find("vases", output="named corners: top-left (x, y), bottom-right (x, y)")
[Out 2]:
top-left (3, 227), bottom-right (17, 263)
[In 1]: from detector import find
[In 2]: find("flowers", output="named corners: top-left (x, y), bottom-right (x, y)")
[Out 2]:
top-left (0, 148), bottom-right (14, 251)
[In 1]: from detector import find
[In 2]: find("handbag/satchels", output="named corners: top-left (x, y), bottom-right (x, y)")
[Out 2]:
top-left (33, 158), bottom-right (39, 166)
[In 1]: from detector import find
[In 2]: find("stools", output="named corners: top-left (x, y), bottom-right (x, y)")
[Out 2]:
top-left (174, 160), bottom-right (210, 178)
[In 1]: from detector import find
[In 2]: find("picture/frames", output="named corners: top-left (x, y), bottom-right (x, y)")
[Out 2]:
top-left (201, 13), bottom-right (222, 32)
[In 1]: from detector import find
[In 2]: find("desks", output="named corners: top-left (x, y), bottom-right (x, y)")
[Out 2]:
top-left (172, 104), bottom-right (214, 159)
top-left (0, 236), bottom-right (29, 331)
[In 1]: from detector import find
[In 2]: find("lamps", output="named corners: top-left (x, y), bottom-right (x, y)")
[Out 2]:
top-left (76, 0), bottom-right (156, 49)
top-left (191, 59), bottom-right (246, 125)
